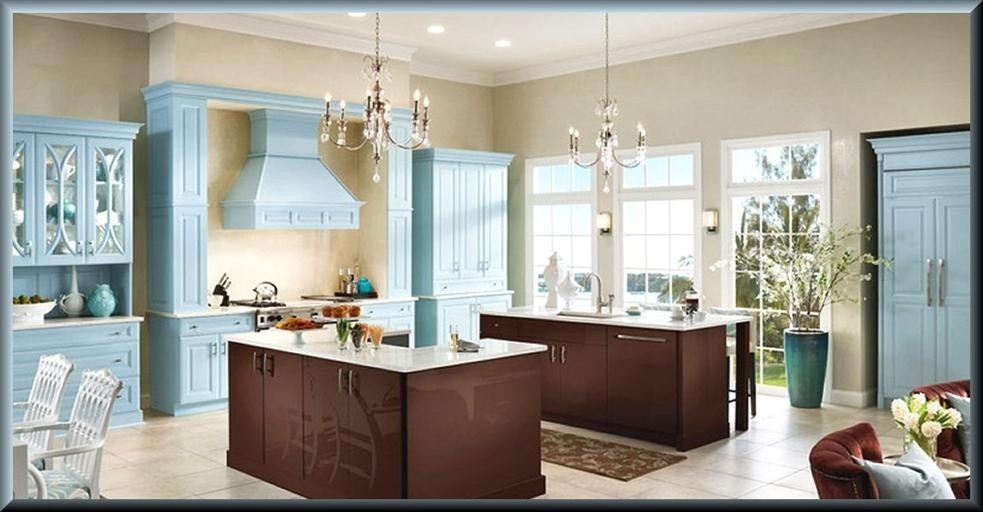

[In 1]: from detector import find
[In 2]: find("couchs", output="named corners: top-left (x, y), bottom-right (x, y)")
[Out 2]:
top-left (910, 379), bottom-right (973, 498)
top-left (809, 424), bottom-right (882, 498)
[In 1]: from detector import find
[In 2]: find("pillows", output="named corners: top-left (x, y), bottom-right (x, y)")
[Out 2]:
top-left (945, 392), bottom-right (971, 464)
top-left (854, 439), bottom-right (956, 503)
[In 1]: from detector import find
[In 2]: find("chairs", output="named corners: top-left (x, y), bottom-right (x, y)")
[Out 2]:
top-left (11, 351), bottom-right (74, 468)
top-left (14, 365), bottom-right (125, 499)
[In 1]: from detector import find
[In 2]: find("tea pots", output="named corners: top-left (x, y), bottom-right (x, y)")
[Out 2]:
top-left (355, 277), bottom-right (374, 294)
top-left (253, 281), bottom-right (278, 303)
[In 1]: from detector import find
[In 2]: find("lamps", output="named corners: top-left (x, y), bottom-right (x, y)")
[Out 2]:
top-left (704, 208), bottom-right (719, 232)
top-left (321, 18), bottom-right (431, 183)
top-left (598, 211), bottom-right (611, 234)
top-left (566, 13), bottom-right (649, 167)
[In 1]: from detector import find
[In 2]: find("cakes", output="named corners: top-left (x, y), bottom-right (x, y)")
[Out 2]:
top-left (349, 305), bottom-right (360, 317)
top-left (340, 304), bottom-right (350, 313)
top-left (322, 305), bottom-right (332, 317)
top-left (332, 306), bottom-right (345, 318)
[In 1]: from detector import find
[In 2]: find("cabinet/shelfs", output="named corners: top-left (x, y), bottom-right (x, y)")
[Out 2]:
top-left (520, 323), bottom-right (606, 427)
top-left (226, 341), bottom-right (302, 497)
top-left (180, 313), bottom-right (255, 414)
top-left (608, 326), bottom-right (678, 445)
top-left (437, 291), bottom-right (515, 351)
top-left (432, 161), bottom-right (513, 297)
top-left (360, 301), bottom-right (413, 334)
top-left (36, 116), bottom-right (144, 265)
top-left (6, 264), bottom-right (144, 432)
top-left (11, 114), bottom-right (36, 265)
top-left (305, 358), bottom-right (405, 504)
top-left (478, 316), bottom-right (519, 342)
top-left (870, 133), bottom-right (970, 409)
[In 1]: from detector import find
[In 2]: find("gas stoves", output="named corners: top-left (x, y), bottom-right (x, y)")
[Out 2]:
top-left (231, 299), bottom-right (335, 310)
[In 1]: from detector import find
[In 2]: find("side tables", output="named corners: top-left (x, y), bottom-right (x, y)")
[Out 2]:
top-left (883, 454), bottom-right (970, 496)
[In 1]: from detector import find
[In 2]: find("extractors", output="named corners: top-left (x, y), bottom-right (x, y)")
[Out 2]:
top-left (220, 108), bottom-right (367, 232)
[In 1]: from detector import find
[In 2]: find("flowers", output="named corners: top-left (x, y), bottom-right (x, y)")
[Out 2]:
top-left (891, 395), bottom-right (963, 460)
top-left (714, 196), bottom-right (894, 331)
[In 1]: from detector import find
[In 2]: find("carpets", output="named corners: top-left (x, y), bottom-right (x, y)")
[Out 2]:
top-left (541, 428), bottom-right (686, 484)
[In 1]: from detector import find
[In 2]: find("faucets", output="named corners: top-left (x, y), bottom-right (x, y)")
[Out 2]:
top-left (584, 272), bottom-right (603, 312)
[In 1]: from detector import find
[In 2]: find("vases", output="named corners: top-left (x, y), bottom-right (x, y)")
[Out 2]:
top-left (780, 326), bottom-right (828, 409)
top-left (902, 431), bottom-right (938, 461)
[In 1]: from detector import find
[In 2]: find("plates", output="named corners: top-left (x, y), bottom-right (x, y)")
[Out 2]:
top-left (669, 315), bottom-right (685, 321)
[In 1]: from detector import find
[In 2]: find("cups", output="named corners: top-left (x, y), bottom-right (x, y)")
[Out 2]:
top-left (337, 317), bottom-right (350, 352)
top-left (360, 323), bottom-right (369, 350)
top-left (370, 326), bottom-right (383, 350)
top-left (350, 323), bottom-right (363, 354)
top-left (672, 306), bottom-right (683, 317)
